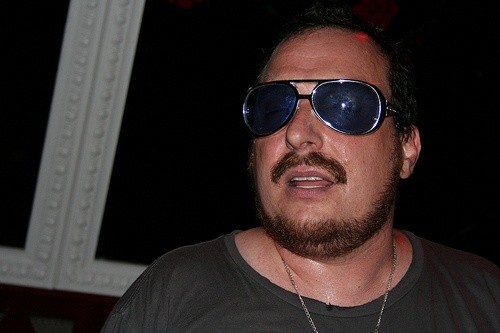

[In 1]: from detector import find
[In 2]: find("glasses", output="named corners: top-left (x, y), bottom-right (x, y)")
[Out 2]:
top-left (241, 78), bottom-right (405, 140)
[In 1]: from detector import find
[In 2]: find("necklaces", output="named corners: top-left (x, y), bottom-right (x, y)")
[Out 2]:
top-left (275, 239), bottom-right (398, 333)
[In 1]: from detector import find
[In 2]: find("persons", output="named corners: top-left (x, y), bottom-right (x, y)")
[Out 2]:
top-left (99, 21), bottom-right (499, 333)
